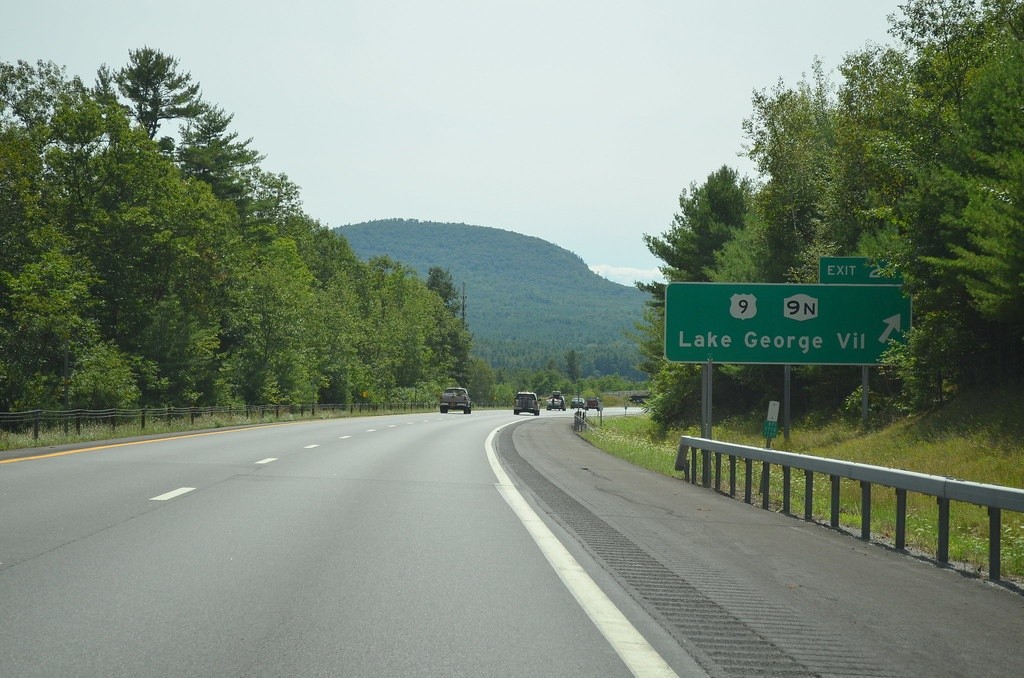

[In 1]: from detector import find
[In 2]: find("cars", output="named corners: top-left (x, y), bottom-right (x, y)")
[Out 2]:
top-left (571, 398), bottom-right (585, 409)
top-left (585, 396), bottom-right (603, 411)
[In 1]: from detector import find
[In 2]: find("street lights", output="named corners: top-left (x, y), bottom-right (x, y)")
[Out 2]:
top-left (460, 282), bottom-right (466, 327)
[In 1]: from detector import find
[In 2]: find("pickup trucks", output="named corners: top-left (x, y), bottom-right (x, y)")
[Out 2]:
top-left (440, 388), bottom-right (471, 414)
top-left (547, 391), bottom-right (566, 411)
top-left (513, 391), bottom-right (541, 415)
